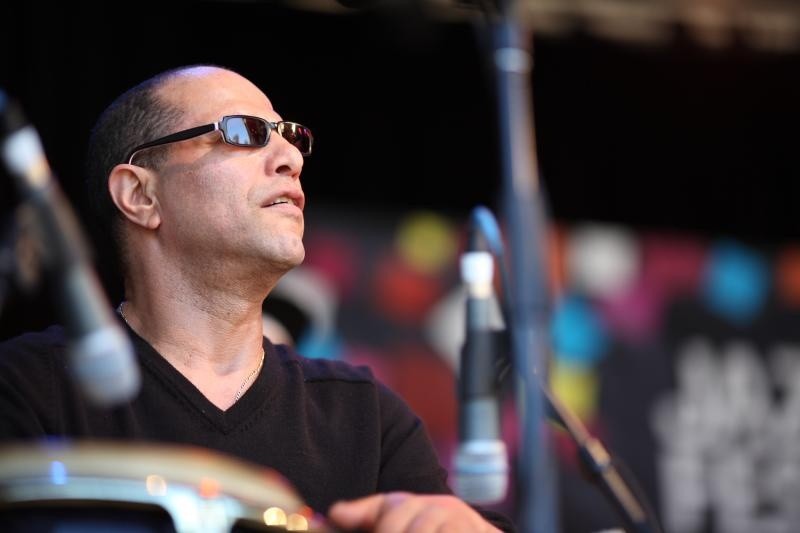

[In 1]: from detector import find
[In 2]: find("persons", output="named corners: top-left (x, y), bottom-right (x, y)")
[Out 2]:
top-left (0, 61), bottom-right (524, 532)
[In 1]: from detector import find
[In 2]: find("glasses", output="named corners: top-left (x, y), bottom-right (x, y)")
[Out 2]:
top-left (124, 113), bottom-right (314, 164)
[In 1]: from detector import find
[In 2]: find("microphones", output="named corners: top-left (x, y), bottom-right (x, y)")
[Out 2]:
top-left (0, 89), bottom-right (141, 407)
top-left (451, 251), bottom-right (510, 505)
